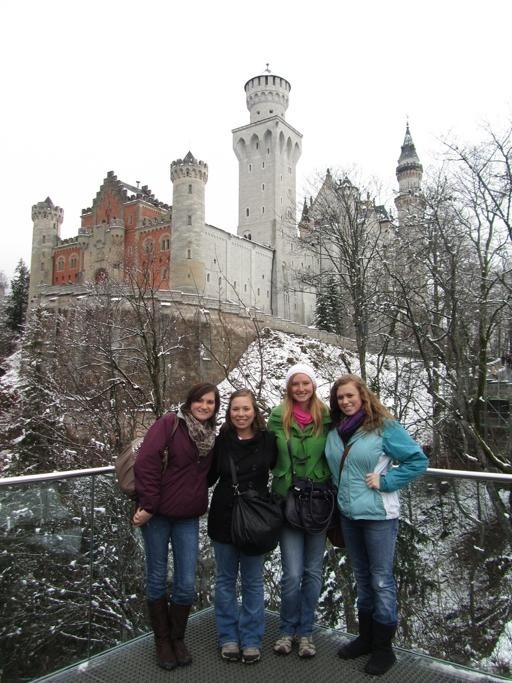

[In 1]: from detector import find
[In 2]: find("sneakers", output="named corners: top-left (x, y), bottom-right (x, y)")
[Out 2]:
top-left (240, 645), bottom-right (262, 665)
top-left (272, 634), bottom-right (295, 656)
top-left (220, 643), bottom-right (241, 662)
top-left (295, 632), bottom-right (317, 658)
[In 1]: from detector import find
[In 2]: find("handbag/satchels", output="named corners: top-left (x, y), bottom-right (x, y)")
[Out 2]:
top-left (283, 475), bottom-right (339, 536)
top-left (231, 483), bottom-right (286, 558)
top-left (114, 435), bottom-right (170, 504)
top-left (327, 498), bottom-right (345, 548)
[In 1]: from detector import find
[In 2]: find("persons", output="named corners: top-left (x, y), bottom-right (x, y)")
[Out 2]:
top-left (206, 388), bottom-right (279, 666)
top-left (264, 360), bottom-right (334, 659)
top-left (323, 372), bottom-right (430, 675)
top-left (131, 382), bottom-right (221, 670)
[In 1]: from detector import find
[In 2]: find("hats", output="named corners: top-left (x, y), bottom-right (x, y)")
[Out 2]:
top-left (285, 362), bottom-right (318, 393)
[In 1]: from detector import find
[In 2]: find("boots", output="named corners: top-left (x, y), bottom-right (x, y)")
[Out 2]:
top-left (146, 598), bottom-right (179, 671)
top-left (337, 613), bottom-right (376, 660)
top-left (168, 599), bottom-right (192, 667)
top-left (364, 620), bottom-right (399, 676)
